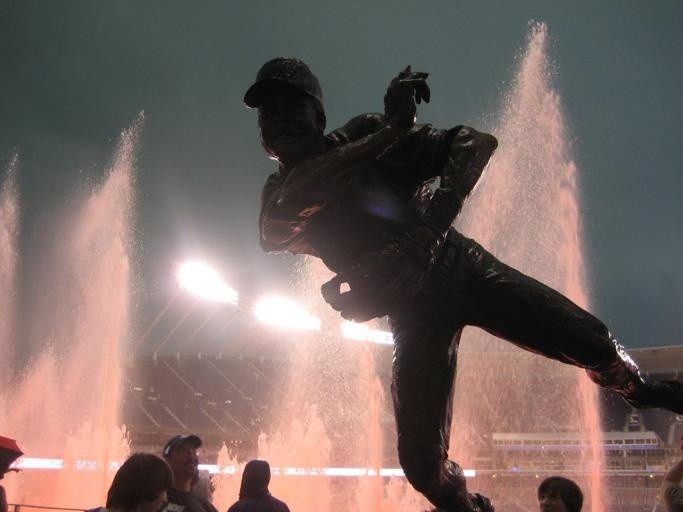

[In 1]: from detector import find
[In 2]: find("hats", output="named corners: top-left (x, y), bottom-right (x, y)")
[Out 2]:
top-left (163, 433), bottom-right (201, 457)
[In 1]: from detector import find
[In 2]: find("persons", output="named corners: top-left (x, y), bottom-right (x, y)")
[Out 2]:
top-left (662, 434), bottom-right (683, 512)
top-left (538, 477), bottom-right (583, 512)
top-left (84, 434), bottom-right (290, 512)
top-left (244, 57), bottom-right (683, 512)
